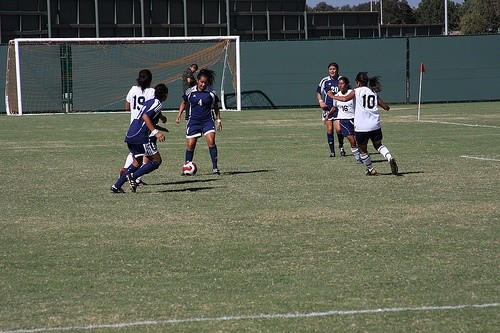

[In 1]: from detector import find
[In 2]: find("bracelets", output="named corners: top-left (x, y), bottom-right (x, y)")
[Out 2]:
top-left (217, 119), bottom-right (221, 123)
top-left (151, 129), bottom-right (158, 135)
top-left (319, 100), bottom-right (323, 103)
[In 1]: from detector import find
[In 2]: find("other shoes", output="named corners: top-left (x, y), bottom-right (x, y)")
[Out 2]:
top-left (110, 184), bottom-right (126, 193)
top-left (354, 152), bottom-right (363, 164)
top-left (126, 172), bottom-right (139, 192)
top-left (329, 152), bottom-right (335, 157)
top-left (340, 151), bottom-right (345, 156)
top-left (364, 167), bottom-right (377, 175)
top-left (212, 168), bottom-right (220, 175)
top-left (389, 157), bottom-right (398, 174)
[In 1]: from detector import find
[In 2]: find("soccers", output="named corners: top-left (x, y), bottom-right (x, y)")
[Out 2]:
top-left (181, 162), bottom-right (197, 176)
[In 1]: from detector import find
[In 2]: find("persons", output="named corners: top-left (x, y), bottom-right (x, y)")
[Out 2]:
top-left (176, 69), bottom-right (223, 176)
top-left (110, 69), bottom-right (168, 193)
top-left (182, 64), bottom-right (198, 120)
top-left (316, 62), bottom-right (399, 176)
